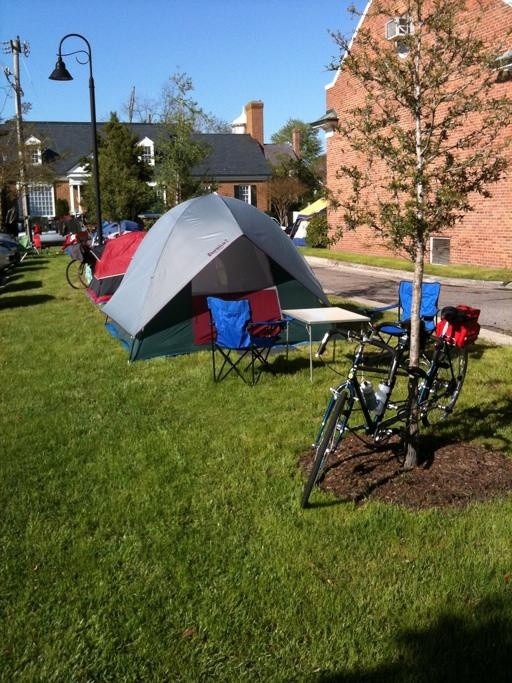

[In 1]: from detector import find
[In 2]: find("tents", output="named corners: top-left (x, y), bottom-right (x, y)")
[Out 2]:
top-left (289, 195), bottom-right (326, 247)
top-left (100, 191), bottom-right (337, 364)
top-left (84, 229), bottom-right (148, 307)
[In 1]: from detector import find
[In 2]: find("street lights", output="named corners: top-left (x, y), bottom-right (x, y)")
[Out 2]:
top-left (47, 31), bottom-right (105, 244)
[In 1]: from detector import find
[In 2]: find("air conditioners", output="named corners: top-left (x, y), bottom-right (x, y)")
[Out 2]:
top-left (385, 17), bottom-right (410, 40)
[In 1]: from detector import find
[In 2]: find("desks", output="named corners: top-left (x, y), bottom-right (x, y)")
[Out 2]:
top-left (282, 306), bottom-right (370, 381)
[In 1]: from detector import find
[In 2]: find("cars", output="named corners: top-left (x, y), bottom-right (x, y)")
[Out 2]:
top-left (0, 229), bottom-right (22, 270)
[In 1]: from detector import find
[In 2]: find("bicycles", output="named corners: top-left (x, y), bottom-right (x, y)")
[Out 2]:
top-left (60, 241), bottom-right (92, 290)
top-left (292, 304), bottom-right (480, 509)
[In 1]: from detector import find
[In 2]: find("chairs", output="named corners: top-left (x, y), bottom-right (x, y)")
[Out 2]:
top-left (207, 295), bottom-right (294, 386)
top-left (364, 276), bottom-right (439, 362)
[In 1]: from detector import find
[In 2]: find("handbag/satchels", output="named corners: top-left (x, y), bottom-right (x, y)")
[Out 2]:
top-left (436, 305), bottom-right (480, 347)
